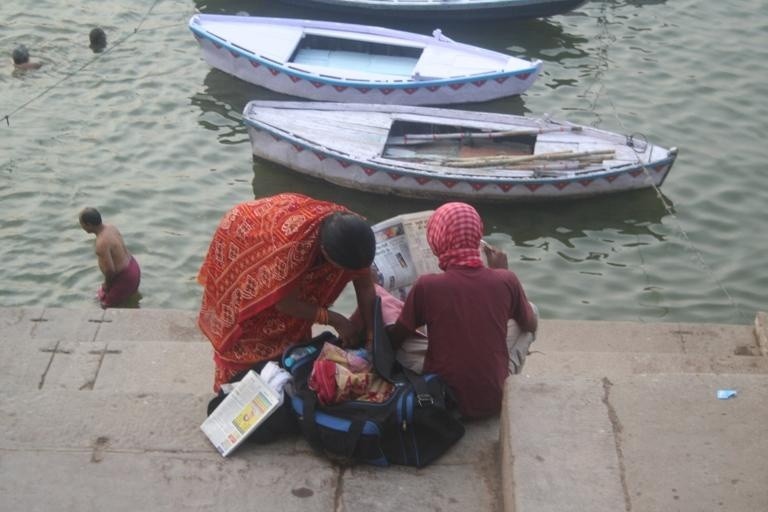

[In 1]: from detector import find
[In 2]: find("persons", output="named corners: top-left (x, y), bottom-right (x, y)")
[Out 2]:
top-left (11, 43), bottom-right (42, 70)
top-left (78, 206), bottom-right (142, 310)
top-left (369, 201), bottom-right (538, 422)
top-left (195, 192), bottom-right (392, 395)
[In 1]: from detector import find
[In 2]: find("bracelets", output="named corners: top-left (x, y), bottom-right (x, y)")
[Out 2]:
top-left (364, 328), bottom-right (372, 345)
top-left (313, 305), bottom-right (328, 325)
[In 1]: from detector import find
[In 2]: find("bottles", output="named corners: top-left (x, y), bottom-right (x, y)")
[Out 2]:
top-left (283, 344), bottom-right (317, 369)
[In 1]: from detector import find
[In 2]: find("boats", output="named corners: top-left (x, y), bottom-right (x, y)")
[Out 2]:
top-left (283, 0), bottom-right (591, 24)
top-left (238, 95), bottom-right (679, 206)
top-left (188, 12), bottom-right (546, 109)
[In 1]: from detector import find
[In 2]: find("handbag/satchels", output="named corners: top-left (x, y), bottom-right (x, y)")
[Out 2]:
top-left (282, 295), bottom-right (466, 470)
